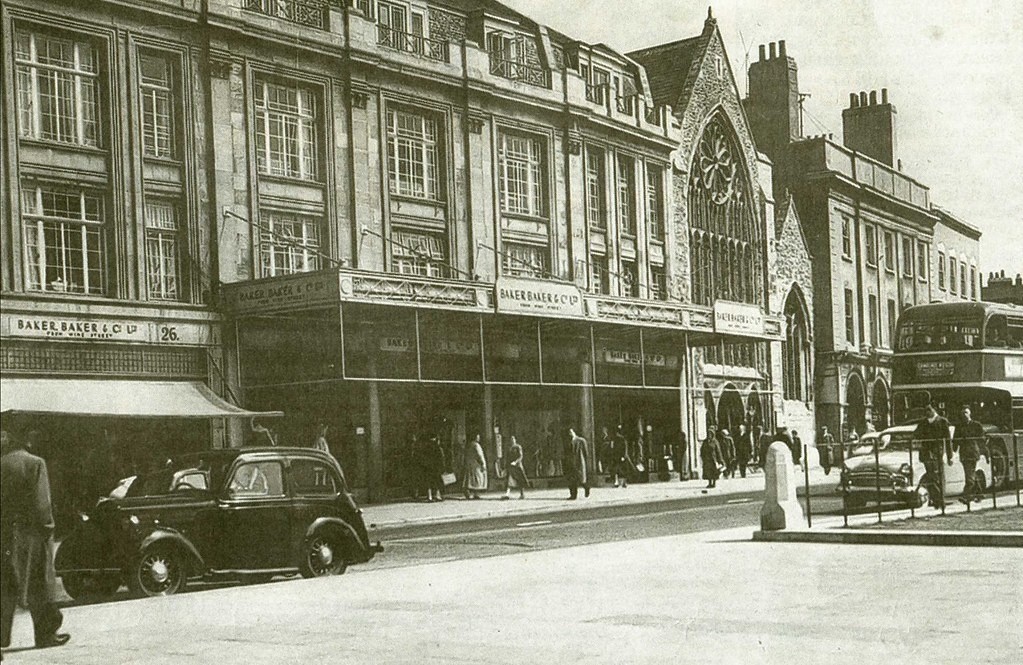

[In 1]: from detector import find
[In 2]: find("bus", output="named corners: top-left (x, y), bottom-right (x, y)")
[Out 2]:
top-left (889, 298), bottom-right (1023, 427)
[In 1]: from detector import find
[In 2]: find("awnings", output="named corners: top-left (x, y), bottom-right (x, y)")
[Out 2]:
top-left (0, 377), bottom-right (284, 420)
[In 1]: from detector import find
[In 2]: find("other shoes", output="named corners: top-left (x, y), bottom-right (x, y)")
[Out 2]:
top-left (567, 495), bottom-right (576, 500)
top-left (428, 498), bottom-right (435, 503)
top-left (959, 497), bottom-right (968, 504)
top-left (584, 486), bottom-right (591, 497)
top-left (36, 633), bottom-right (69, 649)
top-left (501, 496), bottom-right (510, 500)
top-left (435, 496), bottom-right (445, 502)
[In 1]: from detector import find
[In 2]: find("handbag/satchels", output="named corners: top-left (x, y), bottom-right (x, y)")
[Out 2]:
top-left (440, 472), bottom-right (456, 485)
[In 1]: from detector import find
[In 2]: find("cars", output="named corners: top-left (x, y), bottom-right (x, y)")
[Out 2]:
top-left (835, 423), bottom-right (996, 508)
top-left (52, 444), bottom-right (387, 603)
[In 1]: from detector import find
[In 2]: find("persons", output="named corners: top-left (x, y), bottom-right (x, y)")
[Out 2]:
top-left (310, 422), bottom-right (337, 487)
top-left (0, 428), bottom-right (70, 650)
top-left (245, 415), bottom-right (279, 447)
top-left (561, 428), bottom-right (591, 501)
top-left (406, 432), bottom-right (530, 503)
top-left (818, 419), bottom-right (876, 476)
top-left (699, 424), bottom-right (802, 488)
top-left (952, 406), bottom-right (990, 503)
top-left (598, 426), bottom-right (686, 490)
top-left (913, 404), bottom-right (952, 509)
top-left (917, 328), bottom-right (1004, 350)
